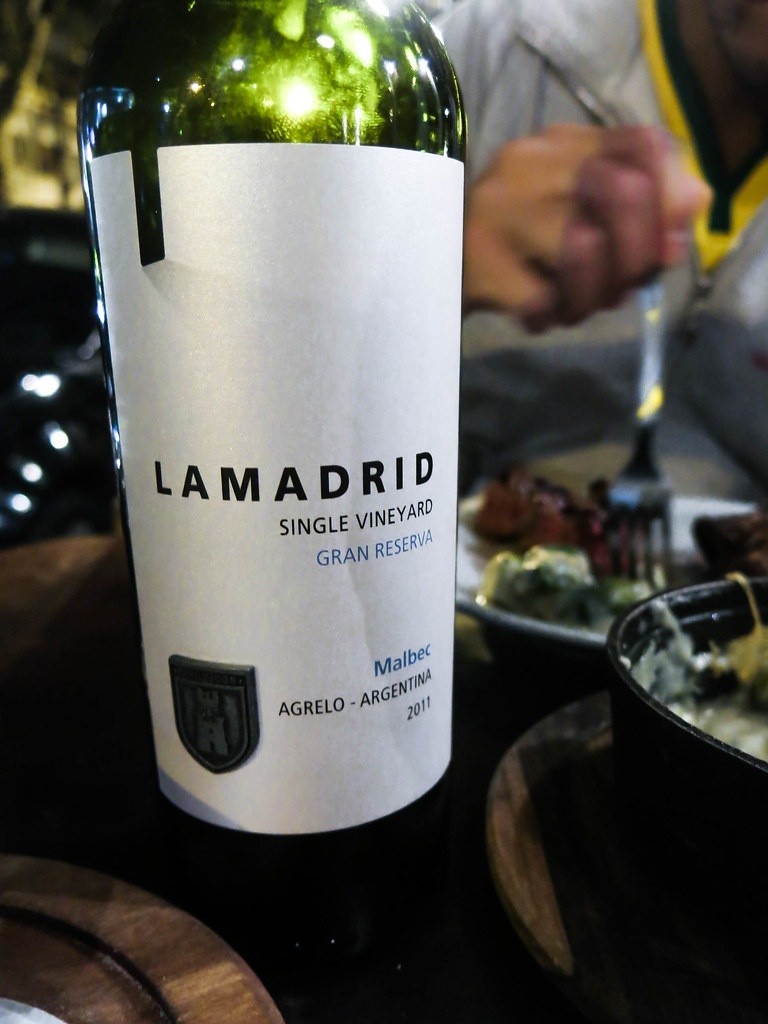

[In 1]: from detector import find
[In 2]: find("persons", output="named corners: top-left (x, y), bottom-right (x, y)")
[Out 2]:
top-left (422, 0), bottom-right (768, 472)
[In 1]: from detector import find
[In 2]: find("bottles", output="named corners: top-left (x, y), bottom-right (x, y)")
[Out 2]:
top-left (77, 0), bottom-right (467, 861)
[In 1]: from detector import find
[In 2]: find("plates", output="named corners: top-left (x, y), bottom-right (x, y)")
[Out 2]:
top-left (0, 850), bottom-right (286, 1023)
top-left (453, 494), bottom-right (768, 656)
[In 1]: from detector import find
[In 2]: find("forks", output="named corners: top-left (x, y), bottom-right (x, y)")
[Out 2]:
top-left (606, 265), bottom-right (676, 589)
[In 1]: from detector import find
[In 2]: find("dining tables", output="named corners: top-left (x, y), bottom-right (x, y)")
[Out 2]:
top-left (0, 426), bottom-right (768, 1024)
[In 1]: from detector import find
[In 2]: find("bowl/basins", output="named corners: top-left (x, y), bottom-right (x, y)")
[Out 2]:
top-left (603, 572), bottom-right (768, 976)
